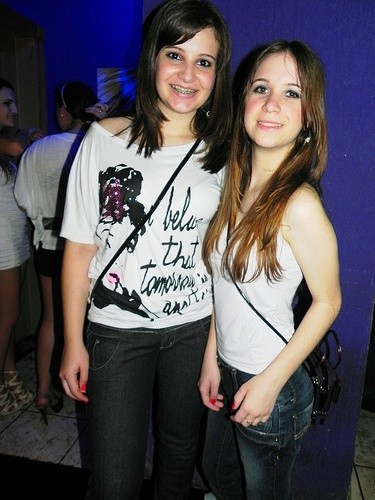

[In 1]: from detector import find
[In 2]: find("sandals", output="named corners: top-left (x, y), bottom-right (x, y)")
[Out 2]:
top-left (4, 370), bottom-right (33, 403)
top-left (0, 384), bottom-right (23, 415)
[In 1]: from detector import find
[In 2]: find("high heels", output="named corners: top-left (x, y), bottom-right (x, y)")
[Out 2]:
top-left (36, 393), bottom-right (63, 424)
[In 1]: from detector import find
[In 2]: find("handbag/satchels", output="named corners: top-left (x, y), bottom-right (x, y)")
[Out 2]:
top-left (302, 329), bottom-right (342, 416)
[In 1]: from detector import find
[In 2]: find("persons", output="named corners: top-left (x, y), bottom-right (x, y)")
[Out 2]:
top-left (198, 39), bottom-right (343, 500)
top-left (0, 81), bottom-right (135, 411)
top-left (60, 0), bottom-right (228, 500)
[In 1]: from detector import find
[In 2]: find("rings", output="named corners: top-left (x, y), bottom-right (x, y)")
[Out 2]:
top-left (244, 417), bottom-right (253, 425)
